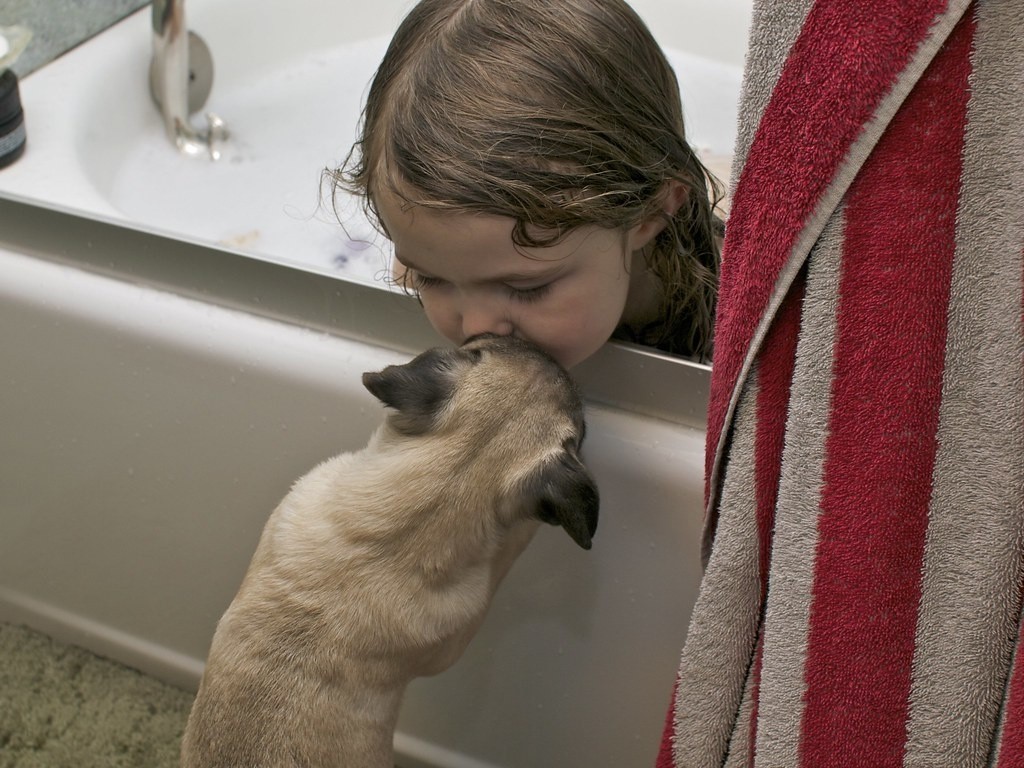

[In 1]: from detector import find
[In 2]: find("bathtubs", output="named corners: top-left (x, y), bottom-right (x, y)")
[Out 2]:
top-left (0, 2), bottom-right (754, 768)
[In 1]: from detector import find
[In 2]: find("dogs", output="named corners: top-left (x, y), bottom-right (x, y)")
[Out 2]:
top-left (173, 332), bottom-right (599, 767)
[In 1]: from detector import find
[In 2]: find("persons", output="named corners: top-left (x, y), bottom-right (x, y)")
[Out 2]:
top-left (319, 1), bottom-right (731, 369)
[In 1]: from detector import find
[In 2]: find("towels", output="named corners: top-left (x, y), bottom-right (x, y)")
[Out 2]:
top-left (654, 0), bottom-right (1024, 768)
